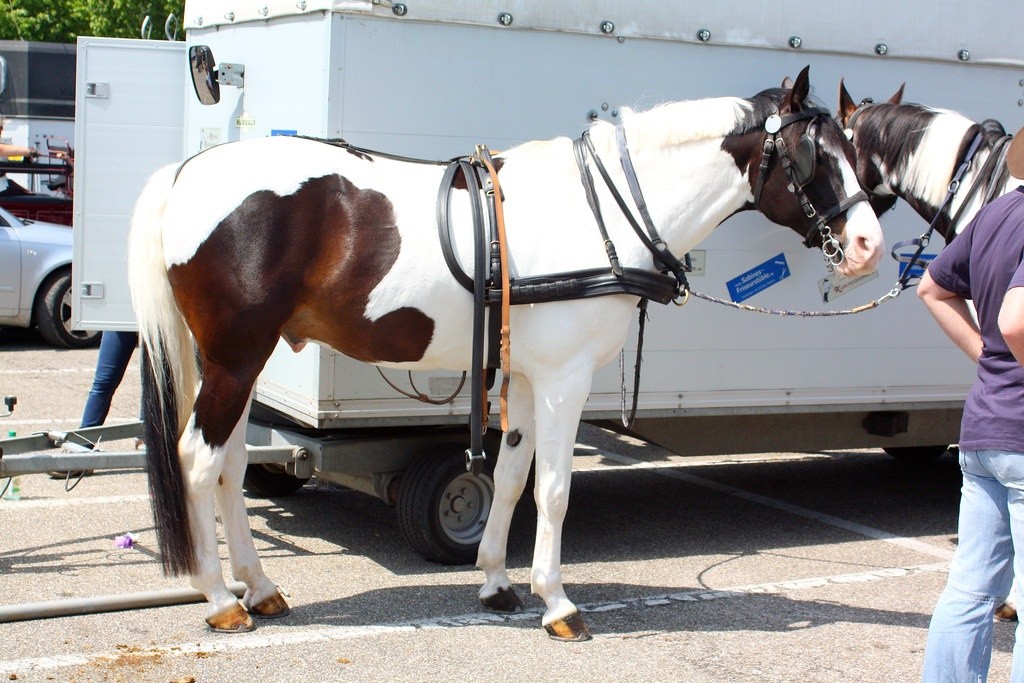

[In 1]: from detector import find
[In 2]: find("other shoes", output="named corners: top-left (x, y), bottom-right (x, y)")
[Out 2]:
top-left (49, 469), bottom-right (94, 478)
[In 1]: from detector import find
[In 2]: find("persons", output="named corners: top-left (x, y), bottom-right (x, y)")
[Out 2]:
top-left (916, 124), bottom-right (1024, 683)
top-left (46, 331), bottom-right (138, 477)
top-left (0, 114), bottom-right (33, 194)
top-left (201, 61), bottom-right (213, 90)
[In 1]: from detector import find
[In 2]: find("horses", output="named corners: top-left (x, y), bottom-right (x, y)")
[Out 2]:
top-left (126, 65), bottom-right (886, 644)
top-left (830, 76), bottom-right (1024, 335)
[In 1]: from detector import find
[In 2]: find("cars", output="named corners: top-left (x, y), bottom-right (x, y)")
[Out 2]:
top-left (1, 162), bottom-right (75, 226)
top-left (0, 204), bottom-right (103, 350)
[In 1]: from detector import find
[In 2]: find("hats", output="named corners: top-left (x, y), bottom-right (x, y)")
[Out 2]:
top-left (1007, 127), bottom-right (1024, 179)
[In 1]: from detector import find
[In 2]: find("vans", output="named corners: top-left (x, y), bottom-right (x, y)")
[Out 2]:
top-left (1, 39), bottom-right (75, 203)
